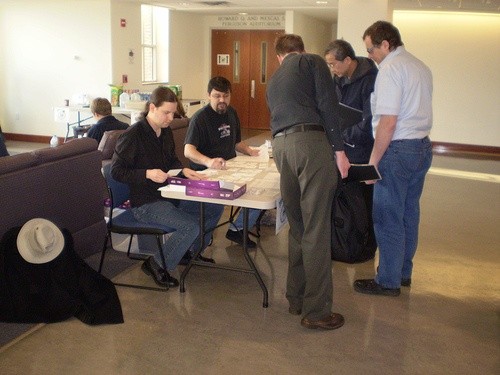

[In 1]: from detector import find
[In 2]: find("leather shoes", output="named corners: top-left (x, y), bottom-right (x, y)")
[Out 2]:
top-left (302, 311), bottom-right (344, 329)
top-left (141, 257), bottom-right (179, 288)
top-left (354, 278), bottom-right (400, 296)
top-left (377, 268), bottom-right (412, 285)
top-left (225, 228), bottom-right (256, 248)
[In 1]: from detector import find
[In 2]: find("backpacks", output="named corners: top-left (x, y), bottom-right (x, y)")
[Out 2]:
top-left (331, 180), bottom-right (377, 263)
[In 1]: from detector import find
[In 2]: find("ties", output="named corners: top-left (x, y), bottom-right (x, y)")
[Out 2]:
top-left (178, 248), bottom-right (216, 266)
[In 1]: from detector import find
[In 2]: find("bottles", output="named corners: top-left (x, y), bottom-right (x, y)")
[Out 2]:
top-left (50, 135), bottom-right (58, 147)
top-left (131, 90), bottom-right (141, 101)
top-left (119, 90), bottom-right (130, 108)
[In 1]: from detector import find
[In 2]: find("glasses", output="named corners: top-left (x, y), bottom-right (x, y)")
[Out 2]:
top-left (366, 42), bottom-right (382, 55)
top-left (326, 56), bottom-right (345, 69)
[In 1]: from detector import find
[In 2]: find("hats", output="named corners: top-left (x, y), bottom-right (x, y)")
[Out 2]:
top-left (17, 218), bottom-right (65, 265)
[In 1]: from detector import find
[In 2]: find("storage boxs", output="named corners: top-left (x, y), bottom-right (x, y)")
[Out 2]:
top-left (182, 179), bottom-right (247, 200)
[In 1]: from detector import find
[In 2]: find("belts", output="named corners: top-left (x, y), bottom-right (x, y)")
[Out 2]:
top-left (275, 124), bottom-right (326, 138)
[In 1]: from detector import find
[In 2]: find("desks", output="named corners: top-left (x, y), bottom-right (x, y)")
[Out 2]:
top-left (161, 154), bottom-right (281, 308)
top-left (66, 104), bottom-right (144, 138)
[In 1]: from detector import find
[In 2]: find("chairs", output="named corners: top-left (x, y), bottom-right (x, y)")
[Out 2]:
top-left (97, 117), bottom-right (191, 292)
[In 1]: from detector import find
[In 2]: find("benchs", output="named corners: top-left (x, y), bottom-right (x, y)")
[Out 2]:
top-left (0, 137), bottom-right (112, 259)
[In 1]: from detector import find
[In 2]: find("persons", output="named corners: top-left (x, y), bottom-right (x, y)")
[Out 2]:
top-left (113, 86), bottom-right (224, 287)
top-left (325, 38), bottom-right (378, 167)
top-left (352, 22), bottom-right (433, 297)
top-left (264, 34), bottom-right (350, 330)
top-left (88, 98), bottom-right (131, 145)
top-left (184, 77), bottom-right (263, 248)
